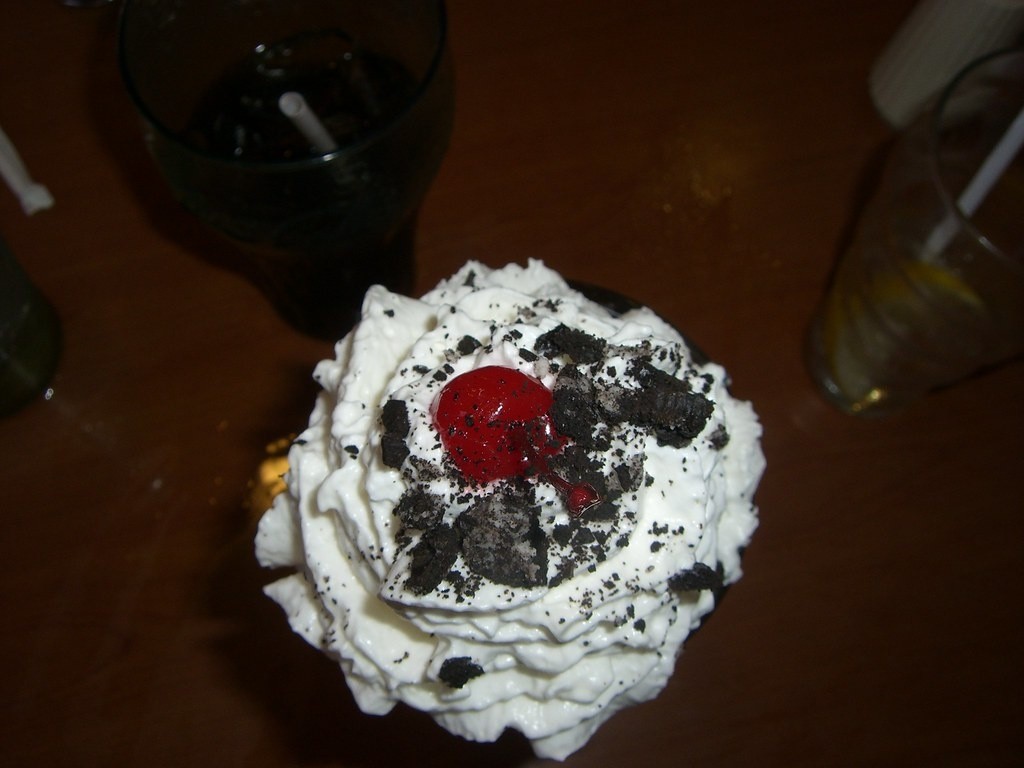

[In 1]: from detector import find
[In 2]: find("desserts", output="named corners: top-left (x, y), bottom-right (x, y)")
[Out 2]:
top-left (256, 260), bottom-right (769, 760)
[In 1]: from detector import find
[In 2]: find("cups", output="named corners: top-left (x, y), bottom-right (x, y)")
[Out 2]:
top-left (867, 0), bottom-right (1024, 131)
top-left (114, 0), bottom-right (454, 339)
top-left (0, 237), bottom-right (65, 419)
top-left (806, 48), bottom-right (1024, 423)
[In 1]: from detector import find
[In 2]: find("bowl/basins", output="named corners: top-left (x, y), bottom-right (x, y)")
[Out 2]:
top-left (565, 278), bottom-right (745, 649)
